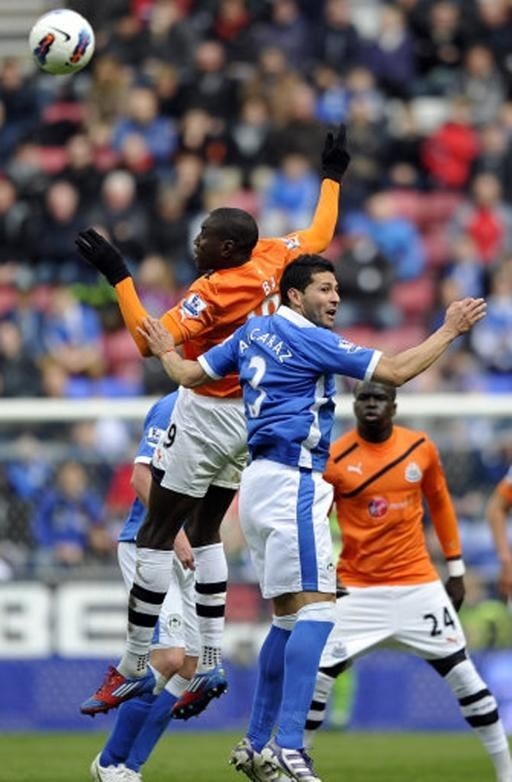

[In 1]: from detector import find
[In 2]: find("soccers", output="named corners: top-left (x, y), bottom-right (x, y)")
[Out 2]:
top-left (29, 9), bottom-right (96, 76)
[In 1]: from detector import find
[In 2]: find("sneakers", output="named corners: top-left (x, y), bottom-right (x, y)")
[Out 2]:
top-left (168, 667), bottom-right (228, 722)
top-left (90, 751), bottom-right (141, 782)
top-left (78, 665), bottom-right (155, 718)
top-left (229, 738), bottom-right (322, 782)
